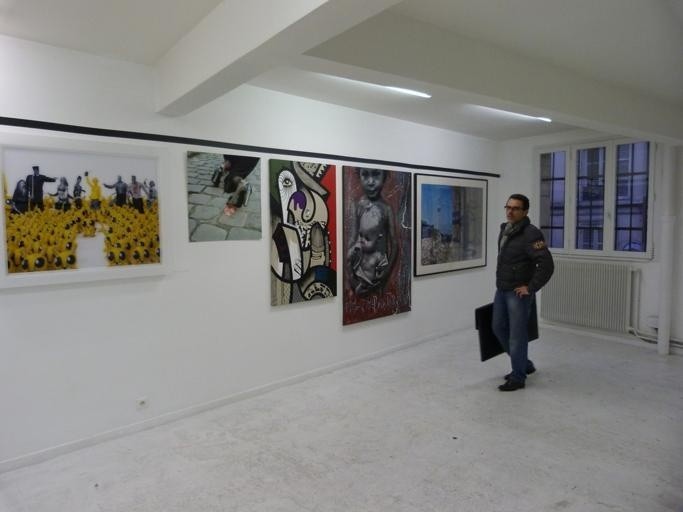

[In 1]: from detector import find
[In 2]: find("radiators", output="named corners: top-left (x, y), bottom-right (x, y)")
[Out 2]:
top-left (539, 256), bottom-right (644, 338)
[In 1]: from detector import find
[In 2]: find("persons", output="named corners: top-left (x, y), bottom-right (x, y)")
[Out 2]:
top-left (211, 151), bottom-right (260, 207)
top-left (346, 204), bottom-right (391, 292)
top-left (492, 193), bottom-right (556, 388)
top-left (12, 164), bottom-right (158, 213)
top-left (346, 166), bottom-right (397, 294)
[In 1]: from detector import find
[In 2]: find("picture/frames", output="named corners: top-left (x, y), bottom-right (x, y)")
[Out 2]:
top-left (0, 132), bottom-right (175, 291)
top-left (411, 174), bottom-right (488, 277)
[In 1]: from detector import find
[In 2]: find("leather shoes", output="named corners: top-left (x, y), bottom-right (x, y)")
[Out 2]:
top-left (505, 360), bottom-right (536, 380)
top-left (499, 373), bottom-right (525, 390)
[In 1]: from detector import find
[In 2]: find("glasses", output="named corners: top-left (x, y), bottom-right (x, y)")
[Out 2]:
top-left (503, 205), bottom-right (520, 210)
